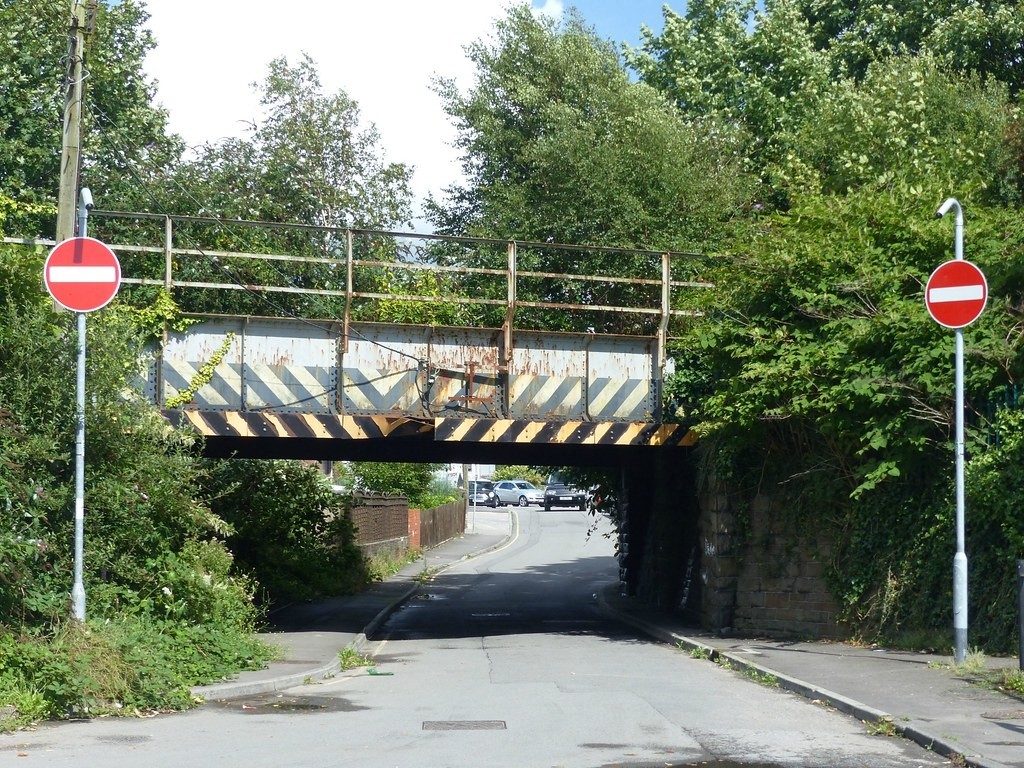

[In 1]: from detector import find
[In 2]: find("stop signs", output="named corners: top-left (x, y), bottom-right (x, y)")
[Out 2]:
top-left (44, 237), bottom-right (121, 312)
top-left (925, 259), bottom-right (989, 328)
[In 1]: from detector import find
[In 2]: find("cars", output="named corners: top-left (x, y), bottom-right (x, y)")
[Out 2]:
top-left (590, 483), bottom-right (617, 513)
top-left (466, 479), bottom-right (546, 508)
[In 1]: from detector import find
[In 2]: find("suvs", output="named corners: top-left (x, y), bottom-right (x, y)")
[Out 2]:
top-left (543, 470), bottom-right (586, 511)
